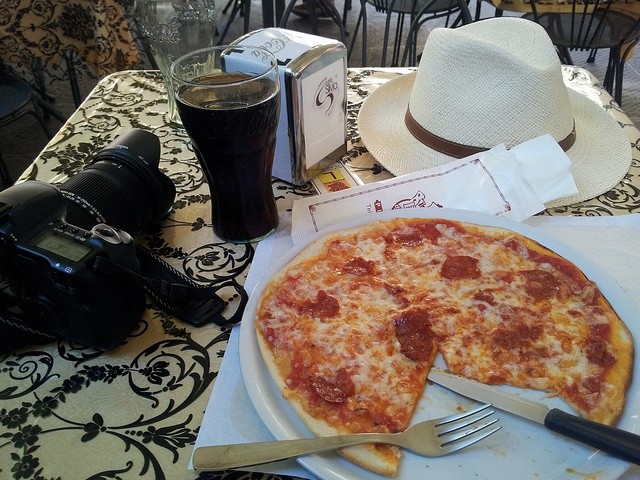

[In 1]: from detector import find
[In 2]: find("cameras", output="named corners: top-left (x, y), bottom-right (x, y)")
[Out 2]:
top-left (0, 129), bottom-right (176, 352)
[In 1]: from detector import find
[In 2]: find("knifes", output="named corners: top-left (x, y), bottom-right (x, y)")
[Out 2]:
top-left (426, 369), bottom-right (640, 465)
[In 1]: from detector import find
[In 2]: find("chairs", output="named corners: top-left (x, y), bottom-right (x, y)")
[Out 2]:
top-left (347, 0), bottom-right (470, 67)
top-left (519, 1), bottom-right (640, 107)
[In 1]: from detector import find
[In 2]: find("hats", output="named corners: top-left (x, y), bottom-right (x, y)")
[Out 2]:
top-left (357, 16), bottom-right (632, 209)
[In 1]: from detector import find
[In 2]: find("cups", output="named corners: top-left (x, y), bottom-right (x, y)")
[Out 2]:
top-left (170, 43), bottom-right (280, 245)
top-left (134, 1), bottom-right (216, 128)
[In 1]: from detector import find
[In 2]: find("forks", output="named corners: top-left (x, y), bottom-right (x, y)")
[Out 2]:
top-left (193, 403), bottom-right (502, 473)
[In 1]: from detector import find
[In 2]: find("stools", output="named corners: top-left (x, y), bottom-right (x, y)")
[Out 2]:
top-left (0, 77), bottom-right (70, 189)
